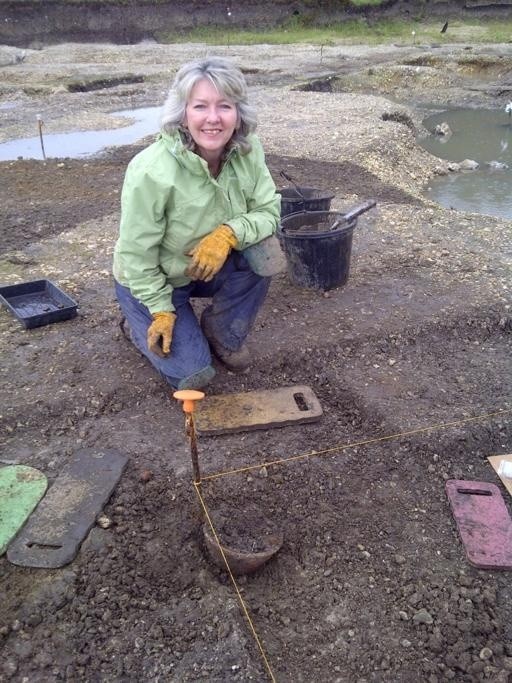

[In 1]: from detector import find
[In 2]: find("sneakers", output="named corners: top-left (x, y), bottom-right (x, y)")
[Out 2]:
top-left (200, 307), bottom-right (253, 372)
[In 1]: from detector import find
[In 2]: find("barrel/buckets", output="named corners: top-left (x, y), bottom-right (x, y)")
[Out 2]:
top-left (277, 210), bottom-right (357, 289)
top-left (276, 189), bottom-right (335, 215)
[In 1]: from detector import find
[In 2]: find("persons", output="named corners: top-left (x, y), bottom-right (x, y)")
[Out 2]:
top-left (113, 57), bottom-right (283, 391)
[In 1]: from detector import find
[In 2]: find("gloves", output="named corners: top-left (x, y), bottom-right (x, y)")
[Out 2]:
top-left (182, 222), bottom-right (239, 283)
top-left (145, 309), bottom-right (178, 358)
